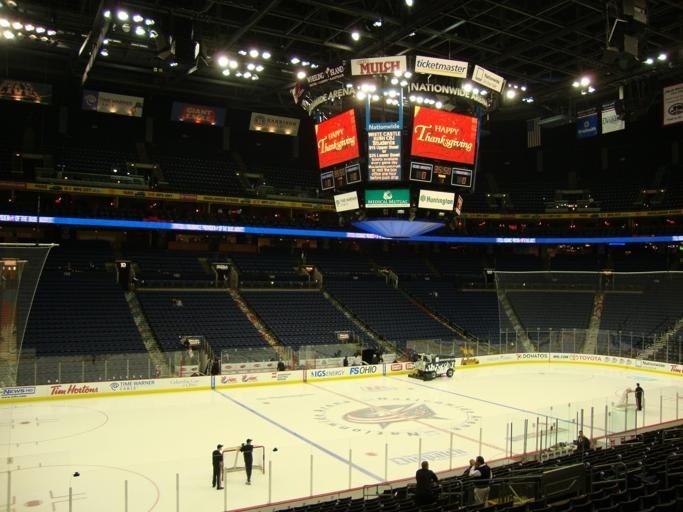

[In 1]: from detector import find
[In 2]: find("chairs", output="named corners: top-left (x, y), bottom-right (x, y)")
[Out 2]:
top-left (275, 424), bottom-right (683, 512)
top-left (17, 236), bottom-right (683, 386)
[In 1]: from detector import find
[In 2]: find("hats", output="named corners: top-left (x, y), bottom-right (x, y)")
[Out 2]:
top-left (217, 444), bottom-right (224, 448)
top-left (246, 439), bottom-right (253, 443)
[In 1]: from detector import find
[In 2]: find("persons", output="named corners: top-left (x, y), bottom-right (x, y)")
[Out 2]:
top-left (276, 359), bottom-right (285, 371)
top-left (211, 443), bottom-right (225, 491)
top-left (469, 456), bottom-right (493, 507)
top-left (342, 355), bottom-right (349, 367)
top-left (462, 460), bottom-right (477, 475)
top-left (575, 431), bottom-right (591, 452)
top-left (240, 437), bottom-right (254, 485)
top-left (414, 460), bottom-right (438, 504)
top-left (211, 358), bottom-right (219, 375)
top-left (633, 383), bottom-right (643, 412)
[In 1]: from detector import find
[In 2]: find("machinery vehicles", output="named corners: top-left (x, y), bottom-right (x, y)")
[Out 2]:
top-left (414, 353), bottom-right (455, 379)
